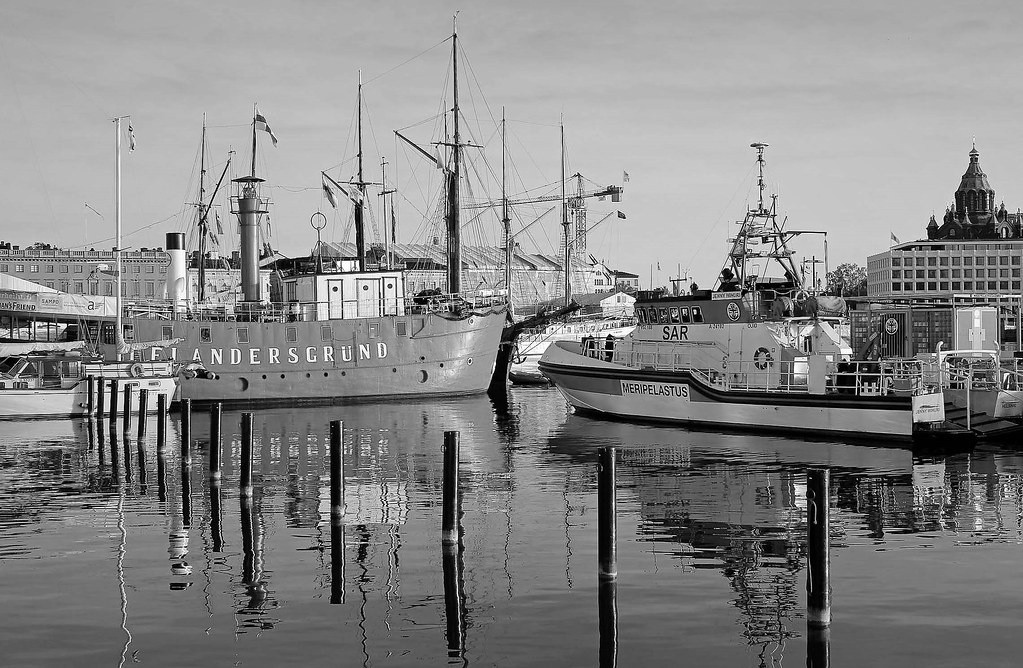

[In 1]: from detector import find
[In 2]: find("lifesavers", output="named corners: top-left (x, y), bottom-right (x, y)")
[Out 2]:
top-left (130, 363), bottom-right (145, 378)
top-left (753, 347), bottom-right (771, 370)
top-left (949, 358), bottom-right (970, 382)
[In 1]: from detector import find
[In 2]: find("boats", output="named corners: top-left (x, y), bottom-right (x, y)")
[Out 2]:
top-left (544, 405), bottom-right (1023, 666)
top-left (508, 370), bottom-right (548, 384)
top-left (62, 171), bottom-right (513, 409)
top-left (0, 111), bottom-right (186, 417)
top-left (54, 391), bottom-right (521, 666)
top-left (531, 140), bottom-right (1022, 445)
top-left (0, 417), bottom-right (175, 666)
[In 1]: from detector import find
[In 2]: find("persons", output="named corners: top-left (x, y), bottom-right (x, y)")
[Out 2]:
top-left (572, 299), bottom-right (577, 315)
top-left (413, 287), bottom-right (443, 309)
top-left (690, 282), bottom-right (699, 294)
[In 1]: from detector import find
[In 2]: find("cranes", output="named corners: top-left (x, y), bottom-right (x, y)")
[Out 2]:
top-left (466, 173), bottom-right (631, 251)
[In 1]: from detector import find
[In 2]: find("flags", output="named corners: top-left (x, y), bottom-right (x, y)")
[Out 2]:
top-left (129, 119), bottom-right (136, 152)
top-left (437, 146), bottom-right (444, 169)
top-left (891, 233), bottom-right (899, 244)
top-left (348, 185), bottom-right (363, 203)
top-left (255, 109), bottom-right (278, 148)
top-left (624, 171), bottom-right (629, 182)
top-left (618, 211), bottom-right (626, 219)
top-left (216, 211), bottom-right (223, 234)
top-left (322, 176), bottom-right (338, 210)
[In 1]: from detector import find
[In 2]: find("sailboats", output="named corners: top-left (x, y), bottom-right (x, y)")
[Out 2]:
top-left (124, 8), bottom-right (641, 374)
